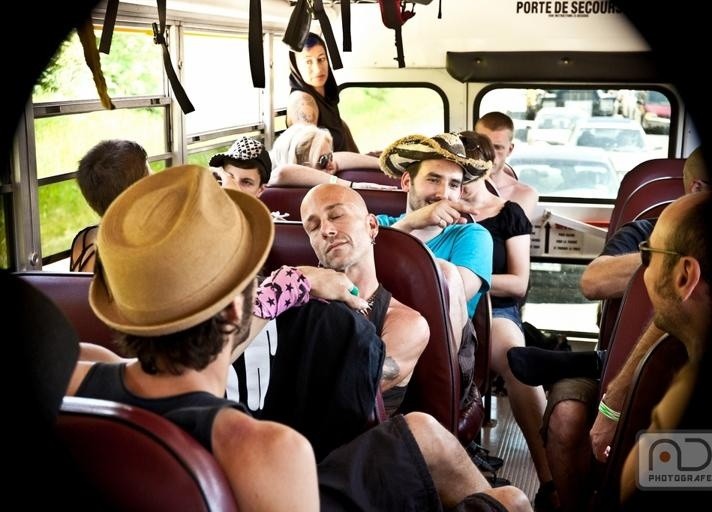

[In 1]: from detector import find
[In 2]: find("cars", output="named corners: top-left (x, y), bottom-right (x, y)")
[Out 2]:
top-left (507, 87), bottom-right (672, 198)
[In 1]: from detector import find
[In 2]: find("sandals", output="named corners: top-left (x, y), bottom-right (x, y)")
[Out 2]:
top-left (465, 441), bottom-right (510, 488)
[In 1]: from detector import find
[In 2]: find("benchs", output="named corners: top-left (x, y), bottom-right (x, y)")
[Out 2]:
top-left (596, 155), bottom-right (689, 509)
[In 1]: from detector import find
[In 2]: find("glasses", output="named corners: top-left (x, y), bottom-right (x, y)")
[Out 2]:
top-left (638, 241), bottom-right (678, 267)
top-left (303, 153), bottom-right (333, 169)
top-left (460, 137), bottom-right (488, 160)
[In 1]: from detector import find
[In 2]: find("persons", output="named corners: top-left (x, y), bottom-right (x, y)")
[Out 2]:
top-left (284, 31), bottom-right (363, 154)
top-left (556, 139), bottom-right (710, 510)
top-left (2, 114), bottom-right (561, 509)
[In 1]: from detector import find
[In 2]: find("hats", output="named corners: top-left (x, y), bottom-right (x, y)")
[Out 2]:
top-left (209, 136), bottom-right (271, 183)
top-left (88, 165), bottom-right (275, 337)
top-left (378, 133), bottom-right (493, 185)
